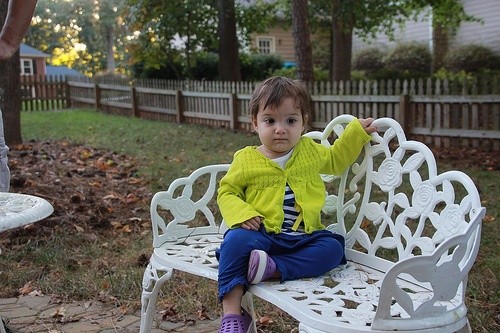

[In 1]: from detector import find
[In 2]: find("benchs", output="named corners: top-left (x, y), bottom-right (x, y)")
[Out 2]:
top-left (137, 115), bottom-right (486, 333)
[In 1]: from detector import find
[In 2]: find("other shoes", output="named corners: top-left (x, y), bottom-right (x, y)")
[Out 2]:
top-left (219, 307), bottom-right (255, 333)
top-left (247, 249), bottom-right (276, 286)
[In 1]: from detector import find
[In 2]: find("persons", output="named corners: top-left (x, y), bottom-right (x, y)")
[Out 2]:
top-left (214, 74), bottom-right (378, 333)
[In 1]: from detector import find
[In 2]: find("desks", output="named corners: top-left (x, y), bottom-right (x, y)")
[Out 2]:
top-left (0, 188), bottom-right (53, 233)
